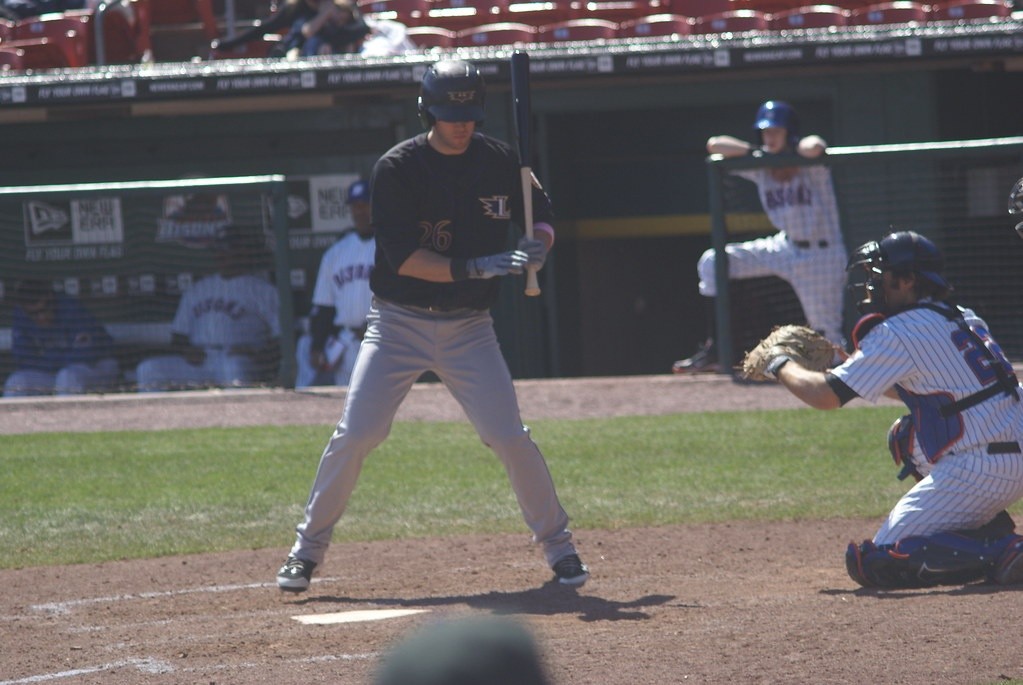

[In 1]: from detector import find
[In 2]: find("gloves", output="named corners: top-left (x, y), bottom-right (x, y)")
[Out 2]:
top-left (519, 237), bottom-right (545, 272)
top-left (465, 246), bottom-right (529, 280)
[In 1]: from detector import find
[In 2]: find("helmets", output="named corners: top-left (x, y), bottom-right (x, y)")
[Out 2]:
top-left (879, 232), bottom-right (954, 294)
top-left (752, 99), bottom-right (799, 135)
top-left (416, 59), bottom-right (486, 132)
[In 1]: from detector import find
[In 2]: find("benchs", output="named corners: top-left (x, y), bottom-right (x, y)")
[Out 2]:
top-left (0, 319), bottom-right (312, 396)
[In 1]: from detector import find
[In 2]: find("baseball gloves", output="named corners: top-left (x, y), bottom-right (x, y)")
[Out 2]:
top-left (740, 322), bottom-right (836, 380)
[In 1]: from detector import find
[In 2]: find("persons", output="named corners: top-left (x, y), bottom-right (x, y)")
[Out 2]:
top-left (673, 101), bottom-right (847, 371)
top-left (137, 225), bottom-right (283, 391)
top-left (5, 279), bottom-right (120, 395)
top-left (744, 231), bottom-right (1023, 587)
top-left (268, 0), bottom-right (372, 57)
top-left (309, 181), bottom-right (373, 386)
top-left (276, 60), bottom-right (587, 591)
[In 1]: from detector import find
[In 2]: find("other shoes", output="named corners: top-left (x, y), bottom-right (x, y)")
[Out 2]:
top-left (994, 534), bottom-right (1023, 585)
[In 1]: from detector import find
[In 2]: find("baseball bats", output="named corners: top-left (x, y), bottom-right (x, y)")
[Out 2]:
top-left (510, 49), bottom-right (543, 296)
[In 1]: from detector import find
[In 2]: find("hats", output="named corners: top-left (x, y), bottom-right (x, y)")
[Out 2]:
top-left (14, 273), bottom-right (51, 306)
top-left (346, 179), bottom-right (372, 202)
top-left (212, 223), bottom-right (244, 254)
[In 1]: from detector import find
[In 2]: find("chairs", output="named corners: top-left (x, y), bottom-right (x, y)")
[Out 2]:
top-left (244, 0), bottom-right (1011, 60)
top-left (0, 0), bottom-right (131, 72)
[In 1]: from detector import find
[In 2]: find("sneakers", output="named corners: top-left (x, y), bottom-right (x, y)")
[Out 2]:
top-left (550, 552), bottom-right (589, 586)
top-left (671, 337), bottom-right (721, 373)
top-left (276, 557), bottom-right (317, 593)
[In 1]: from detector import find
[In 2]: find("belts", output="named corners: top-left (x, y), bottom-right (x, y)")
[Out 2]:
top-left (784, 235), bottom-right (826, 248)
top-left (428, 305), bottom-right (446, 313)
top-left (334, 326), bottom-right (366, 340)
top-left (949, 441), bottom-right (1022, 456)
